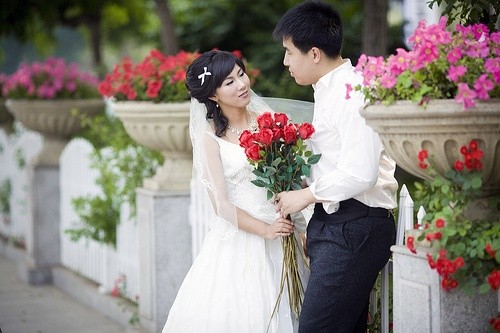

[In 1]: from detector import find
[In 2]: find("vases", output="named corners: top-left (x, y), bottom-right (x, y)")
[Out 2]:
top-left (109, 101), bottom-right (194, 193)
top-left (357, 99), bottom-right (500, 218)
top-left (2, 98), bottom-right (104, 165)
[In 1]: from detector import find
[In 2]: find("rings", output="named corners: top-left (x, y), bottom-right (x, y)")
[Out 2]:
top-left (281, 208), bottom-right (284, 212)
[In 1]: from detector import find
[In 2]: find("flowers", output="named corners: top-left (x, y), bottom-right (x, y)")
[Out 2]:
top-left (97, 49), bottom-right (262, 106)
top-left (345, 14), bottom-right (500, 333)
top-left (238, 112), bottom-right (323, 333)
top-left (0, 56), bottom-right (99, 100)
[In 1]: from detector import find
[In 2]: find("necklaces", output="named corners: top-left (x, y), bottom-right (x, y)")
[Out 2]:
top-left (227, 111), bottom-right (259, 136)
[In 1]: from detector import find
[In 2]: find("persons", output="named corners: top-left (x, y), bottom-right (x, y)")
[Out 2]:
top-left (161, 49), bottom-right (315, 333)
top-left (270, 1), bottom-right (399, 333)
